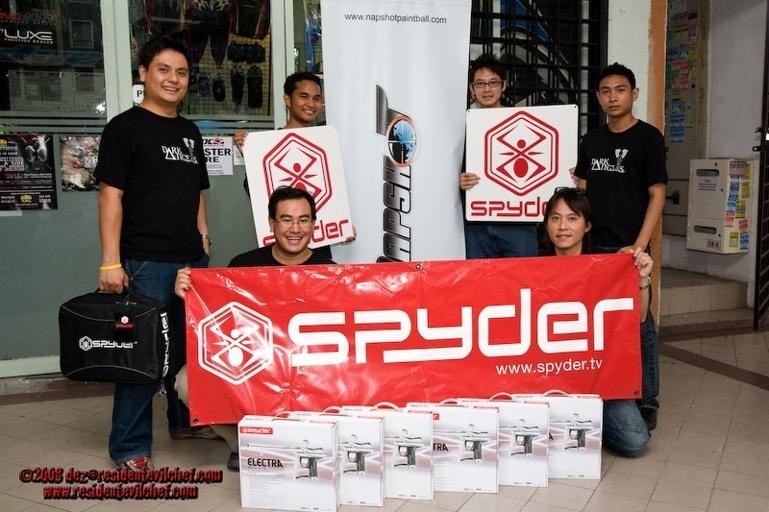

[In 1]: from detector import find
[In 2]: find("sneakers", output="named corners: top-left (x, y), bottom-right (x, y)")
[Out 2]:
top-left (173, 427), bottom-right (221, 439)
top-left (638, 407), bottom-right (657, 430)
top-left (119, 457), bottom-right (154, 474)
top-left (228, 453), bottom-right (240, 470)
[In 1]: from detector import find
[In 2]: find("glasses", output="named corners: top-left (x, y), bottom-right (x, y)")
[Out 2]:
top-left (274, 216), bottom-right (310, 227)
top-left (555, 187), bottom-right (587, 194)
top-left (475, 81), bottom-right (501, 88)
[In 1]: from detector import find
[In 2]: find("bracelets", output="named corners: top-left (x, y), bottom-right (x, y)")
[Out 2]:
top-left (201, 234), bottom-right (212, 247)
top-left (99, 263), bottom-right (121, 270)
top-left (640, 277), bottom-right (653, 290)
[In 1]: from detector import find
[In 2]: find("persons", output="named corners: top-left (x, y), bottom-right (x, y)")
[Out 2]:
top-left (459, 54), bottom-right (576, 258)
top-left (234, 73), bottom-right (358, 245)
top-left (574, 62), bottom-right (668, 431)
top-left (171, 185), bottom-right (337, 473)
top-left (91, 38), bottom-right (225, 483)
top-left (543, 187), bottom-right (656, 459)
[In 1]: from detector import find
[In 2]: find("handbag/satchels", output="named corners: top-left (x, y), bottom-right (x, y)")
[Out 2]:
top-left (59, 280), bottom-right (177, 384)
